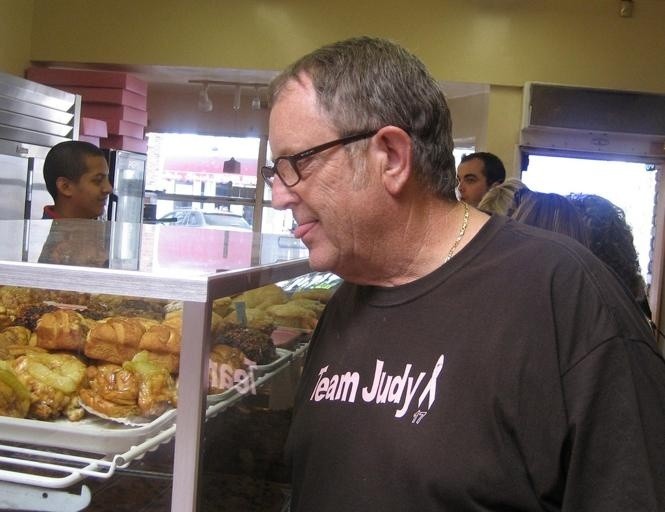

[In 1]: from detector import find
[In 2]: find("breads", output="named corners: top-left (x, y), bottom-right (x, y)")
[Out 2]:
top-left (0, 281), bottom-right (333, 421)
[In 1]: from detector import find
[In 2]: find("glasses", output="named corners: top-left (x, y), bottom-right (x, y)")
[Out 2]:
top-left (261, 127), bottom-right (412, 188)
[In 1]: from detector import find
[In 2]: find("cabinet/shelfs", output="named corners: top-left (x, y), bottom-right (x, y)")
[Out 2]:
top-left (0, 221), bottom-right (346, 512)
top-left (101, 149), bottom-right (147, 270)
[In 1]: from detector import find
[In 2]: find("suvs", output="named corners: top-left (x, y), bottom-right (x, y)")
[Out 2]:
top-left (140, 207), bottom-right (254, 271)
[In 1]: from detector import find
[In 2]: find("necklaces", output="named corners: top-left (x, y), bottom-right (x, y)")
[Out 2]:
top-left (444, 200), bottom-right (472, 264)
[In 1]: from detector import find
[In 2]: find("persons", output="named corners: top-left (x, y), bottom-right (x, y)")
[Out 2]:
top-left (566, 193), bottom-right (653, 326)
top-left (475, 177), bottom-right (530, 222)
top-left (38, 221), bottom-right (113, 272)
top-left (454, 150), bottom-right (505, 209)
top-left (258, 36), bottom-right (665, 510)
top-left (509, 191), bottom-right (592, 254)
top-left (30, 141), bottom-right (114, 221)
